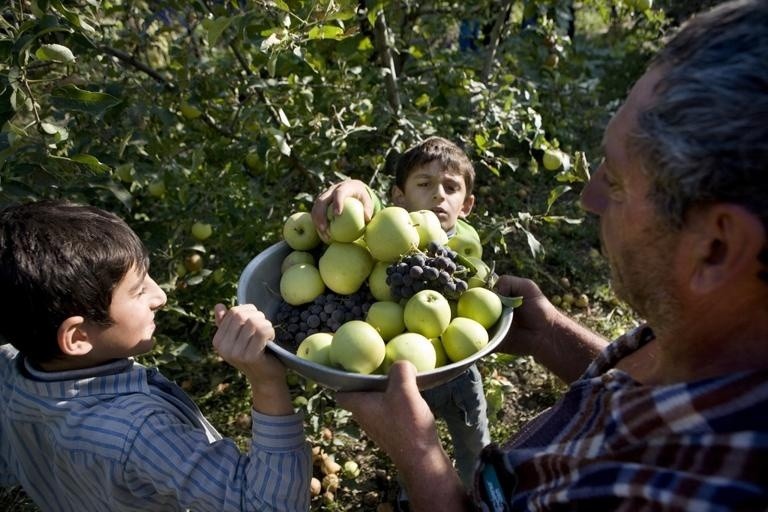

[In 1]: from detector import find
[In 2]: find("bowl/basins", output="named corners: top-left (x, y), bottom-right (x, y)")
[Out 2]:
top-left (236, 237), bottom-right (516, 396)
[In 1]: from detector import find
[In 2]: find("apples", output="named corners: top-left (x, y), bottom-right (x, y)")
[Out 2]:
top-left (118, 33), bottom-right (588, 506)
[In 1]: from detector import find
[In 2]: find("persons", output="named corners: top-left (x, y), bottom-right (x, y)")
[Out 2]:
top-left (330, 1), bottom-right (767, 512)
top-left (310, 134), bottom-right (494, 512)
top-left (0, 199), bottom-right (316, 512)
